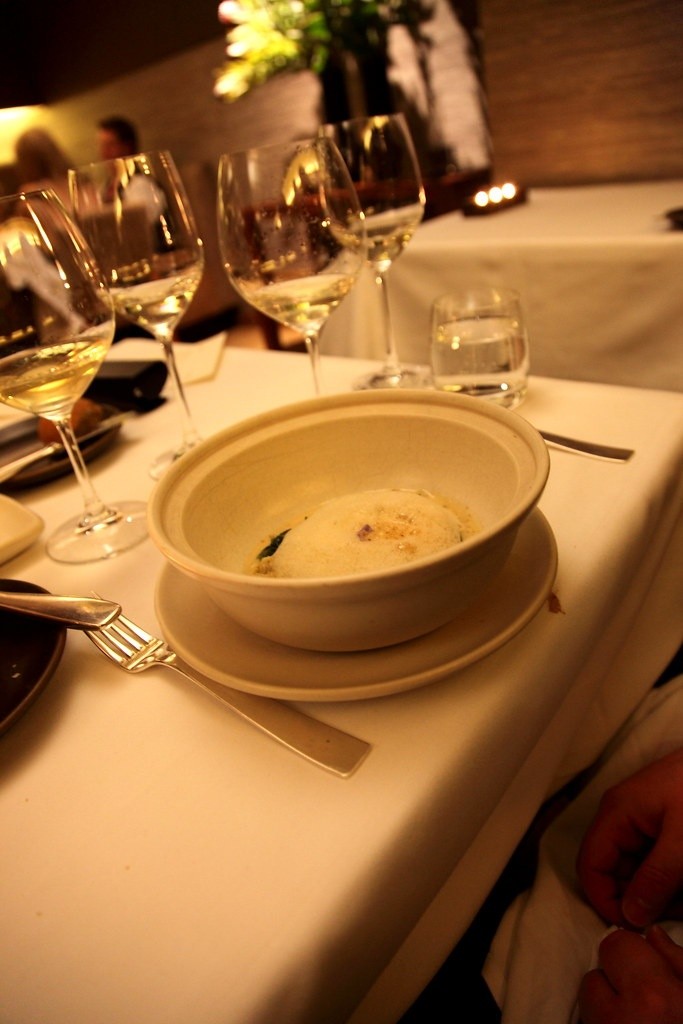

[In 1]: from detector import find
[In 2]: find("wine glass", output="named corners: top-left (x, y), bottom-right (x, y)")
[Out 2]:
top-left (0, 186), bottom-right (150, 563)
top-left (318, 113), bottom-right (433, 390)
top-left (67, 150), bottom-right (204, 480)
top-left (216, 137), bottom-right (366, 395)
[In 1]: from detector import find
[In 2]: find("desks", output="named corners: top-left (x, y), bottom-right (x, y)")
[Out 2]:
top-left (0, 348), bottom-right (683, 1024)
top-left (310, 179), bottom-right (683, 391)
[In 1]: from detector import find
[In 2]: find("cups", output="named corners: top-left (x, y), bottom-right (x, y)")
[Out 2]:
top-left (427, 285), bottom-right (529, 411)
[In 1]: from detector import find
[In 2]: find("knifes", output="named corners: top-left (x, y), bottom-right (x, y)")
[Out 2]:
top-left (0, 411), bottom-right (136, 484)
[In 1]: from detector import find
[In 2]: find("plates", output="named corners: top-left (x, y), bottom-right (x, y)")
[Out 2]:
top-left (0, 579), bottom-right (66, 736)
top-left (151, 508), bottom-right (559, 700)
top-left (0, 404), bottom-right (123, 488)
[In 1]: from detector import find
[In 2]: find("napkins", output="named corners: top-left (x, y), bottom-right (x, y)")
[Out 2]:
top-left (107, 330), bottom-right (227, 386)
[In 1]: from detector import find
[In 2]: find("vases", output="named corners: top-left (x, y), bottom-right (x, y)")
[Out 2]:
top-left (316, 45), bottom-right (452, 182)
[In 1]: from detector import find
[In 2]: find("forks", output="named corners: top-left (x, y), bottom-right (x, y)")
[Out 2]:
top-left (76, 590), bottom-right (372, 778)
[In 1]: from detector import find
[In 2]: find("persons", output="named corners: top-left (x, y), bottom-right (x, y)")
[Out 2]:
top-left (309, 0), bottom-right (498, 270)
top-left (403, 673), bottom-right (683, 1024)
top-left (15, 129), bottom-right (99, 350)
top-left (102, 111), bottom-right (194, 276)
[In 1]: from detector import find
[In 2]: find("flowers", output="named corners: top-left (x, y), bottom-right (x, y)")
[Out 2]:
top-left (212, 0), bottom-right (433, 106)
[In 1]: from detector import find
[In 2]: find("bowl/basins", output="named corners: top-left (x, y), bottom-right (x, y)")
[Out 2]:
top-left (147, 388), bottom-right (550, 651)
